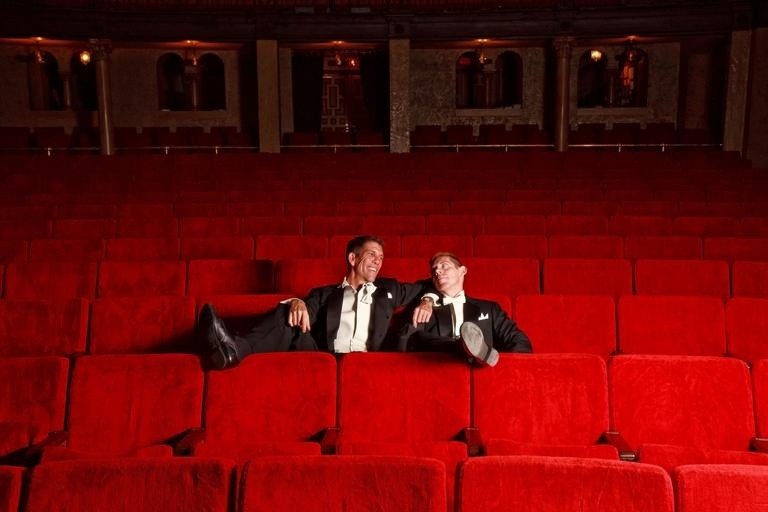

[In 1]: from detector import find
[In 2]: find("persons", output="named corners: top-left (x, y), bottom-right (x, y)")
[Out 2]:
top-left (395, 251), bottom-right (531, 367)
top-left (198, 235), bottom-right (443, 368)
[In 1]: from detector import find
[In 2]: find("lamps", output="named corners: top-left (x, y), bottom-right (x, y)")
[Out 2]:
top-left (590, 48), bottom-right (603, 62)
top-left (475, 38), bottom-right (489, 64)
top-left (80, 48), bottom-right (91, 65)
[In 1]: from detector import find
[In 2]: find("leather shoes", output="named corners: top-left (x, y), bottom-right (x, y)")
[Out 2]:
top-left (199, 304), bottom-right (238, 369)
top-left (460, 321), bottom-right (499, 368)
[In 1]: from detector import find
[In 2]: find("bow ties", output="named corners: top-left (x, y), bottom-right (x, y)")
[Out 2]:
top-left (443, 295), bottom-right (466, 305)
top-left (361, 286), bottom-right (377, 304)
